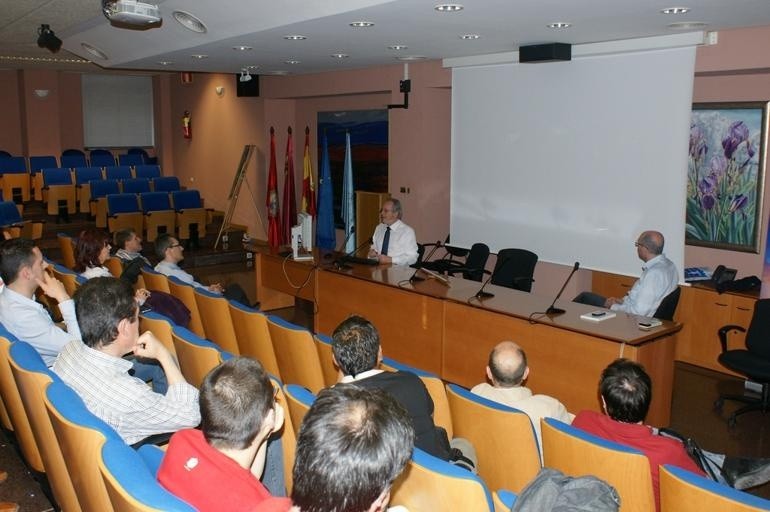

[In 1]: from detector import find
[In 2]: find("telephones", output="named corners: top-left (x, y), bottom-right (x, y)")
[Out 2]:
top-left (712, 265), bottom-right (738, 286)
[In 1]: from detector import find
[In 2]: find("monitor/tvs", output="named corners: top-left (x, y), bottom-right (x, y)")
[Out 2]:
top-left (290, 211), bottom-right (314, 262)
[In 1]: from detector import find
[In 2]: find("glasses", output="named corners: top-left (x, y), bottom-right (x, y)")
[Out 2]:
top-left (634, 242), bottom-right (649, 249)
top-left (378, 207), bottom-right (393, 213)
top-left (168, 242), bottom-right (182, 248)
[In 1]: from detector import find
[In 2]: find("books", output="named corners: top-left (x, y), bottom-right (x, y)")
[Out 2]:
top-left (580, 309), bottom-right (617, 322)
top-left (685, 266), bottom-right (711, 282)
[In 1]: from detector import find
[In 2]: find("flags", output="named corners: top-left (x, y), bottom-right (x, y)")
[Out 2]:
top-left (340, 128), bottom-right (356, 258)
top-left (300, 126), bottom-right (317, 225)
top-left (284, 126), bottom-right (297, 247)
top-left (318, 129), bottom-right (336, 250)
top-left (264, 126), bottom-right (281, 251)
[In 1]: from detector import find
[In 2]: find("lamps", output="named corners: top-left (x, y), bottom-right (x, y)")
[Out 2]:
top-left (35, 24), bottom-right (63, 53)
top-left (517, 41), bottom-right (572, 65)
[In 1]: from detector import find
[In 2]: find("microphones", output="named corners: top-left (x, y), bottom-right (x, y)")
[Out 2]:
top-left (408, 239), bottom-right (442, 282)
top-left (547, 262), bottom-right (579, 315)
top-left (324, 226), bottom-right (356, 260)
top-left (335, 236), bottom-right (374, 271)
top-left (475, 255), bottom-right (515, 298)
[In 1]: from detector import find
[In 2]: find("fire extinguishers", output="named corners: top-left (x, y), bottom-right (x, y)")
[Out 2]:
top-left (182, 111), bottom-right (192, 138)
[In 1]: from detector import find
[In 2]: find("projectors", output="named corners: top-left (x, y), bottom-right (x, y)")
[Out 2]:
top-left (103, 0), bottom-right (162, 27)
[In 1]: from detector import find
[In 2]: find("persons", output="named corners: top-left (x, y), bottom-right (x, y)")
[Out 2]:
top-left (295, 383), bottom-right (415, 512)
top-left (114, 228), bottom-right (151, 269)
top-left (331, 315), bottom-right (480, 478)
top-left (472, 342), bottom-right (577, 470)
top-left (52, 277), bottom-right (204, 447)
top-left (1, 237), bottom-right (82, 374)
top-left (571, 230), bottom-right (680, 317)
top-left (569, 357), bottom-right (770, 512)
top-left (76, 227), bottom-right (191, 329)
top-left (152, 233), bottom-right (260, 313)
top-left (365, 199), bottom-right (419, 267)
top-left (158, 356), bottom-right (292, 511)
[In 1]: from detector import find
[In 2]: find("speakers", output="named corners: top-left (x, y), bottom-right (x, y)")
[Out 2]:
top-left (519, 43), bottom-right (571, 64)
top-left (236, 73), bottom-right (260, 97)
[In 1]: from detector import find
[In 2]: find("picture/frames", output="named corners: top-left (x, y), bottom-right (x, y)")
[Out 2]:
top-left (684, 101), bottom-right (769, 254)
